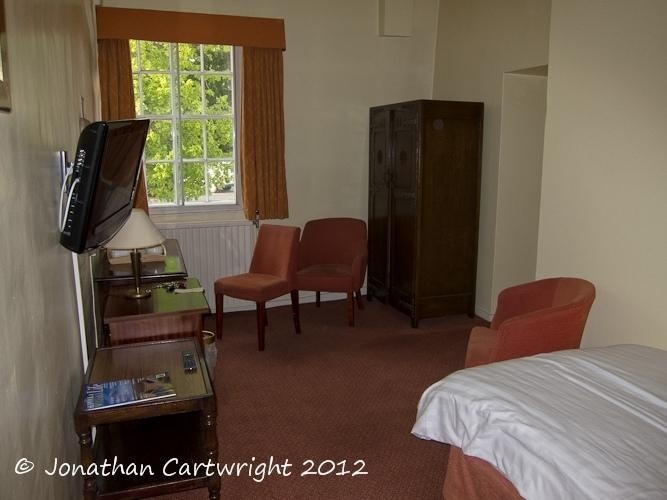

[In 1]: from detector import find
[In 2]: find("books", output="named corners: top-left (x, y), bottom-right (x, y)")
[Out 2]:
top-left (82, 372), bottom-right (176, 411)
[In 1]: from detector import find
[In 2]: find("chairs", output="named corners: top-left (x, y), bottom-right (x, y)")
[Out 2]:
top-left (296, 216), bottom-right (370, 330)
top-left (465, 275), bottom-right (597, 368)
top-left (213, 221), bottom-right (305, 354)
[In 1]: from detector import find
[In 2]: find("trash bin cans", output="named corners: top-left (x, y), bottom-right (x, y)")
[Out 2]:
top-left (202, 330), bottom-right (218, 383)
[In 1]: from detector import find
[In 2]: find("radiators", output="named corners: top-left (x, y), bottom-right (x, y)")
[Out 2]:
top-left (111, 219), bottom-right (258, 312)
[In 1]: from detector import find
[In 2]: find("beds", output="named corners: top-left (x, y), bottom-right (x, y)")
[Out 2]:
top-left (413, 343), bottom-right (667, 500)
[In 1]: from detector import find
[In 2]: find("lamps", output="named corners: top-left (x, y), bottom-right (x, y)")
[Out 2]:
top-left (100, 206), bottom-right (165, 299)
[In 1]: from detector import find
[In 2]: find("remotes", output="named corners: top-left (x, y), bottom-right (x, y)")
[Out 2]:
top-left (181, 351), bottom-right (197, 373)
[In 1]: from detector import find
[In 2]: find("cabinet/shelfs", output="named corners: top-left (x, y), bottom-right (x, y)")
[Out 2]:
top-left (366, 98), bottom-right (486, 329)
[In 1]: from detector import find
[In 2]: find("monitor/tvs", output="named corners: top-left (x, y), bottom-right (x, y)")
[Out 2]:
top-left (59, 118), bottom-right (150, 255)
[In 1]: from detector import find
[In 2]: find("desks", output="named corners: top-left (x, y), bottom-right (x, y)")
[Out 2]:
top-left (94, 237), bottom-right (187, 308)
top-left (103, 277), bottom-right (212, 348)
top-left (72, 334), bottom-right (221, 500)
